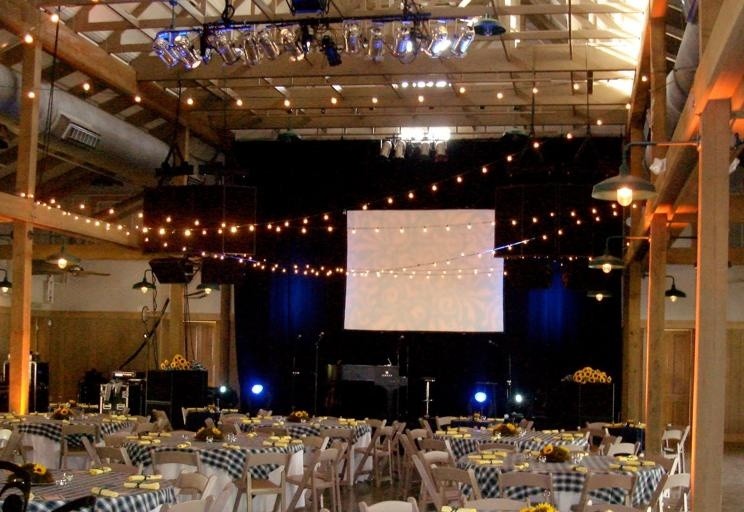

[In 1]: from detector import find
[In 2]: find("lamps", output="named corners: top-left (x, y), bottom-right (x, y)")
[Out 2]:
top-left (469, 8), bottom-right (506, 41)
top-left (196, 279), bottom-right (221, 296)
top-left (587, 232), bottom-right (651, 277)
top-left (589, 133), bottom-right (706, 213)
top-left (0, 268), bottom-right (12, 294)
top-left (147, 0), bottom-right (475, 74)
top-left (585, 284), bottom-right (613, 303)
top-left (132, 268), bottom-right (161, 296)
top-left (378, 132), bottom-right (450, 161)
top-left (641, 265), bottom-right (691, 306)
top-left (22, 229), bottom-right (85, 267)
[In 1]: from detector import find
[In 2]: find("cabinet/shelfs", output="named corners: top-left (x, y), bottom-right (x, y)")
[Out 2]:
top-left (96, 378), bottom-right (144, 414)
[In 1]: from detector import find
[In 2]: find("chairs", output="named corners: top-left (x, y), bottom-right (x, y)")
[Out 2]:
top-left (2, 412), bottom-right (691, 512)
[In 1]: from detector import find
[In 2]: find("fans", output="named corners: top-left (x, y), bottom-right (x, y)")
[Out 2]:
top-left (30, 262), bottom-right (113, 282)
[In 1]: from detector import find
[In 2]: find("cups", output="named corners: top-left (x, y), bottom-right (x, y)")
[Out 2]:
top-left (481, 416), bottom-right (485, 421)
top-left (183, 433), bottom-right (188, 441)
top-left (569, 451), bottom-right (580, 464)
top-left (224, 432), bottom-right (239, 444)
top-left (300, 418), bottom-right (306, 424)
top-left (495, 430), bottom-right (501, 438)
top-left (55, 469), bottom-right (73, 489)
top-left (539, 456), bottom-right (546, 464)
top-left (206, 435), bottom-right (212, 443)
top-left (480, 426), bottom-right (486, 434)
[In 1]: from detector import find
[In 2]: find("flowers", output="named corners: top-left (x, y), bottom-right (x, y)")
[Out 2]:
top-left (160, 351), bottom-right (187, 370)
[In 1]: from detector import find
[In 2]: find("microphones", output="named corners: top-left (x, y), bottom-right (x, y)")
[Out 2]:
top-left (295, 334), bottom-right (302, 341)
top-left (488, 340), bottom-right (499, 348)
top-left (396, 335), bottom-right (404, 344)
top-left (317, 331), bottom-right (325, 340)
